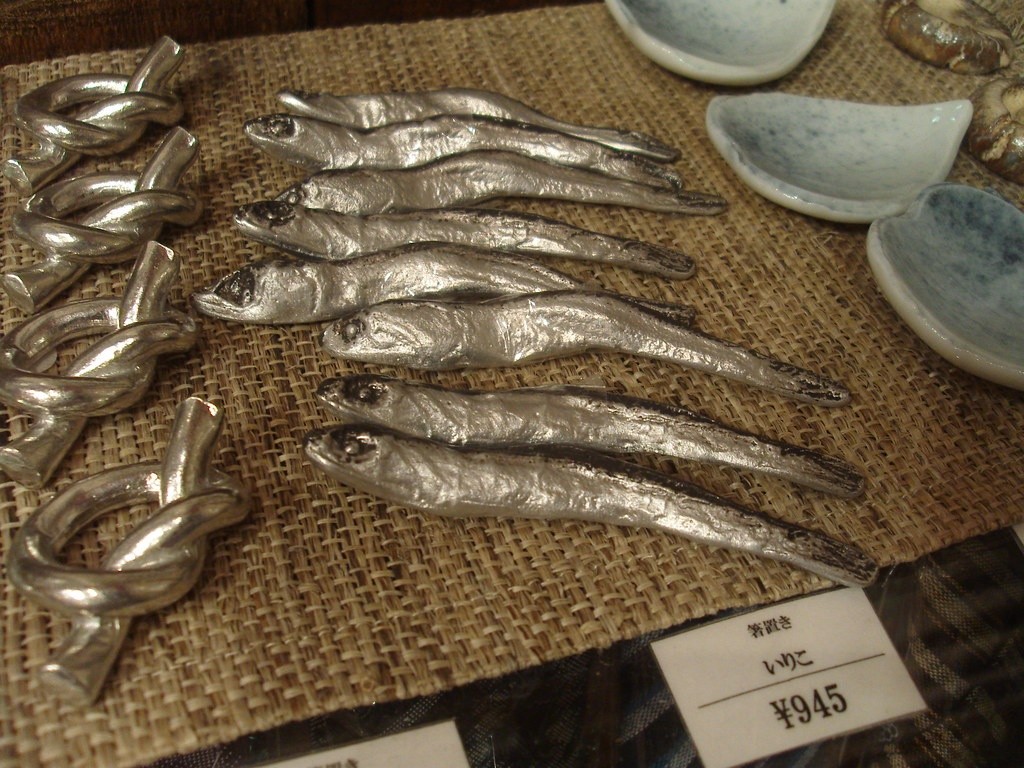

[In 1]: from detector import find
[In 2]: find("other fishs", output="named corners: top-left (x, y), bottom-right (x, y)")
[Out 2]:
top-left (187, 87), bottom-right (881, 589)
